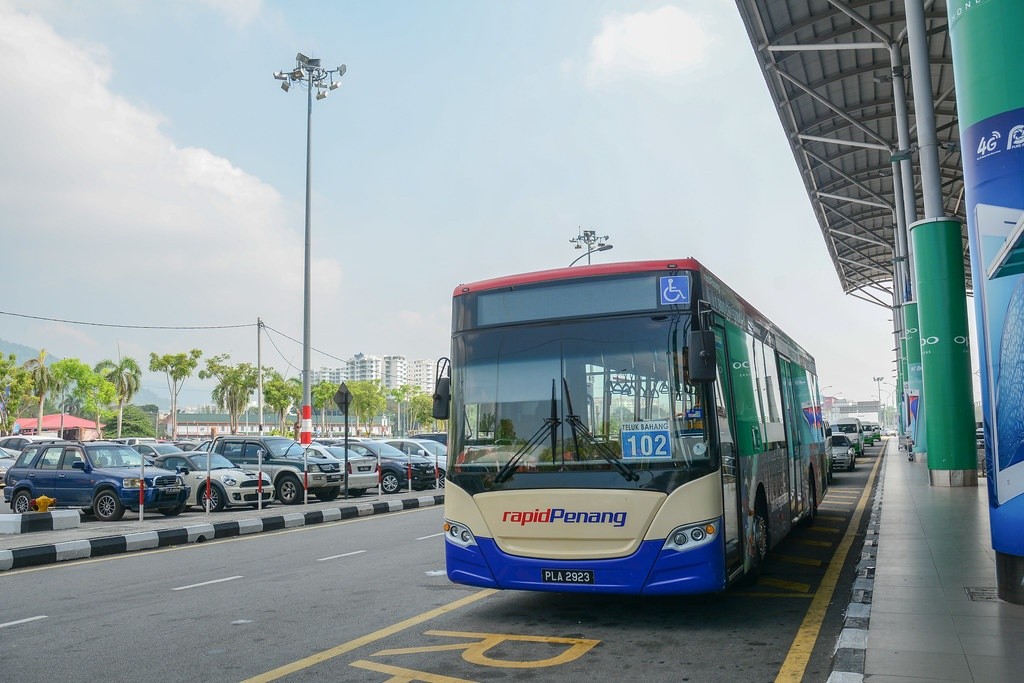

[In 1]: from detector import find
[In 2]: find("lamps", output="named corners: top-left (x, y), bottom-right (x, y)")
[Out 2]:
top-left (892, 305), bottom-right (901, 309)
top-left (883, 226), bottom-right (894, 230)
top-left (892, 330), bottom-right (900, 378)
top-left (873, 74), bottom-right (888, 83)
top-left (878, 171), bottom-right (890, 176)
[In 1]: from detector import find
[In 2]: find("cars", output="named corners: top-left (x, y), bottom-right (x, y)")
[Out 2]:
top-left (881, 428), bottom-right (896, 436)
top-left (208, 436), bottom-right (345, 506)
top-left (832, 417), bottom-right (866, 456)
top-left (307, 437), bottom-right (446, 495)
top-left (0, 435), bottom-right (189, 521)
top-left (126, 442), bottom-right (277, 508)
top-left (860, 421), bottom-right (882, 447)
top-left (827, 436), bottom-right (858, 471)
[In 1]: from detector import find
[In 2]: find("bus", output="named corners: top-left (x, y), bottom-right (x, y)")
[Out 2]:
top-left (430, 257), bottom-right (834, 616)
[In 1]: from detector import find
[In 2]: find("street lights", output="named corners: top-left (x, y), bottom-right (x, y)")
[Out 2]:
top-left (274, 52), bottom-right (350, 445)
top-left (873, 376), bottom-right (887, 410)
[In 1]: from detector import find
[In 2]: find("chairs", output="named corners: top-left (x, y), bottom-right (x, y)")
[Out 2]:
top-left (98, 457), bottom-right (108, 467)
top-left (591, 434), bottom-right (622, 460)
